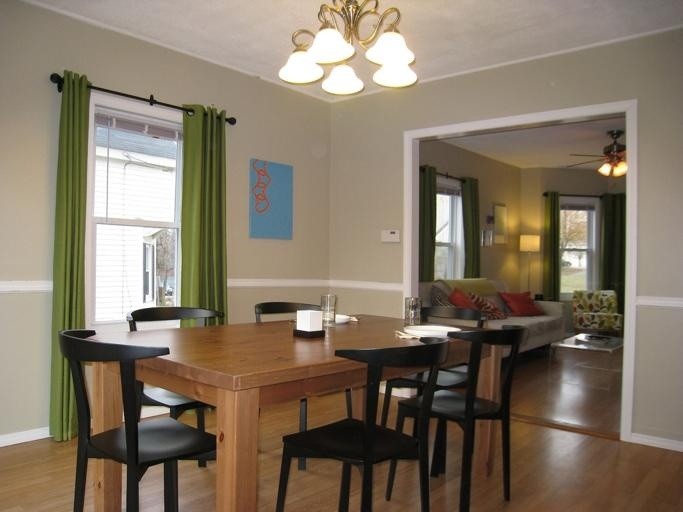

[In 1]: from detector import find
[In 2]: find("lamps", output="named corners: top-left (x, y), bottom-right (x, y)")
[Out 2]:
top-left (597, 159), bottom-right (627, 178)
top-left (518, 233), bottom-right (541, 289)
top-left (276, 0), bottom-right (419, 97)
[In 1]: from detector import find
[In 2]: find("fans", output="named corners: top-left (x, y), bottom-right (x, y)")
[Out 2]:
top-left (566, 129), bottom-right (625, 170)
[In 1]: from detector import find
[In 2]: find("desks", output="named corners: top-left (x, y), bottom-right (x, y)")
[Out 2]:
top-left (66, 311), bottom-right (511, 509)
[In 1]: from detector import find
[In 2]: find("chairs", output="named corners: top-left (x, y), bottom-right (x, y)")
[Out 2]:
top-left (56, 324), bottom-right (217, 512)
top-left (254, 300), bottom-right (360, 475)
top-left (377, 295), bottom-right (488, 477)
top-left (273, 335), bottom-right (454, 511)
top-left (121, 303), bottom-right (231, 471)
top-left (382, 323), bottom-right (526, 503)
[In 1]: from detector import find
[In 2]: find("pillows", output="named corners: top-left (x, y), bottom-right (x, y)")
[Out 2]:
top-left (447, 287), bottom-right (480, 311)
top-left (496, 287), bottom-right (546, 317)
top-left (467, 289), bottom-right (506, 319)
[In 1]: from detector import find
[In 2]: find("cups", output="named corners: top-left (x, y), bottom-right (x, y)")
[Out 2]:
top-left (403, 296), bottom-right (421, 327)
top-left (321, 293), bottom-right (338, 326)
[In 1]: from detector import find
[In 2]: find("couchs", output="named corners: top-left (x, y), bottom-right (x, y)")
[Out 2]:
top-left (571, 288), bottom-right (623, 337)
top-left (417, 277), bottom-right (568, 376)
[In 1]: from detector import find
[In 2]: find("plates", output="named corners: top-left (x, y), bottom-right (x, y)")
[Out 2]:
top-left (324, 312), bottom-right (351, 325)
top-left (402, 326), bottom-right (446, 340)
